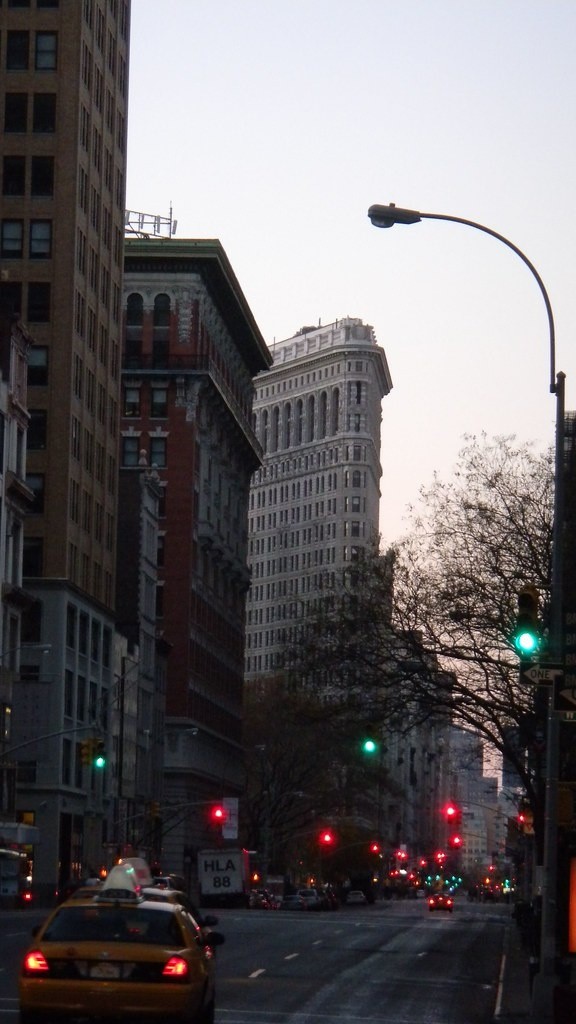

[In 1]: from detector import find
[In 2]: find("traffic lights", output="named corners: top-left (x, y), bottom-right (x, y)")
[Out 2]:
top-left (516, 584), bottom-right (541, 656)
top-left (212, 802), bottom-right (223, 825)
top-left (93, 748), bottom-right (108, 770)
top-left (447, 805), bottom-right (460, 826)
top-left (364, 739), bottom-right (384, 756)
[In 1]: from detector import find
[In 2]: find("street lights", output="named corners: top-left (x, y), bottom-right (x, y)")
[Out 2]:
top-left (266, 830), bottom-right (334, 892)
top-left (325, 839), bottom-right (435, 898)
top-left (369, 203), bottom-right (567, 1020)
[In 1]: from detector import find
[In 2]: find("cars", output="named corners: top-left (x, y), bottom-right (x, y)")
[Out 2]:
top-left (250, 887), bottom-right (365, 909)
top-left (18, 857), bottom-right (224, 1024)
top-left (428, 892), bottom-right (451, 911)
